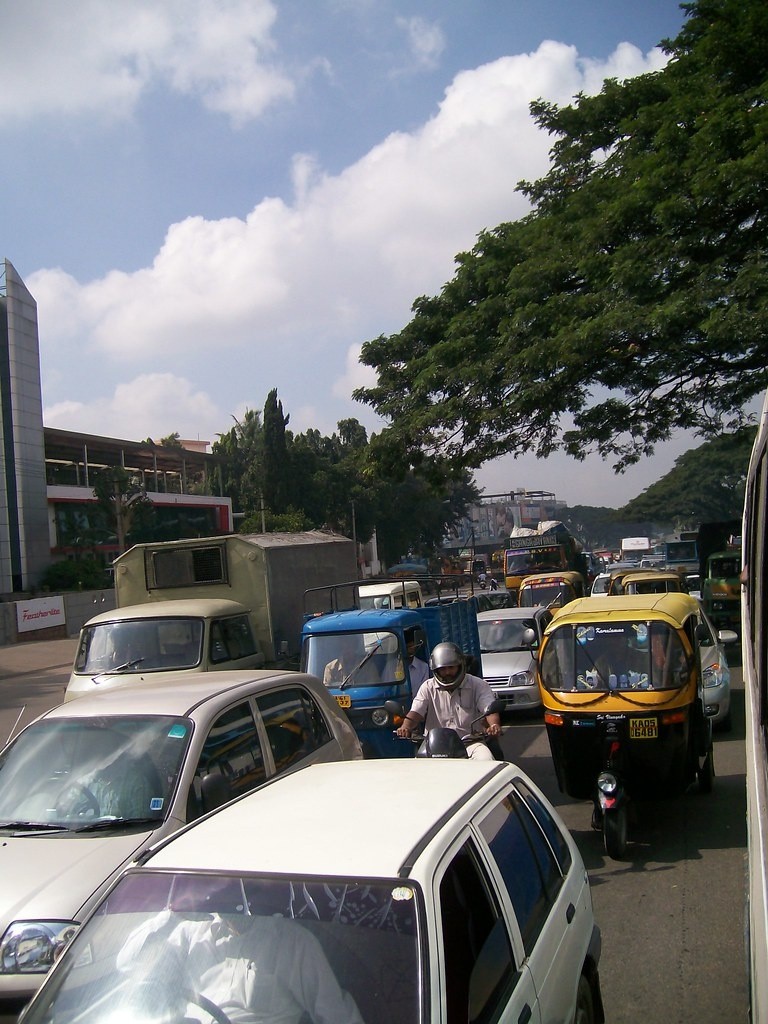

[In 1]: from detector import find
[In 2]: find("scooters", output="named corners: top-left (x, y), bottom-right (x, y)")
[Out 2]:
top-left (476, 577), bottom-right (487, 589)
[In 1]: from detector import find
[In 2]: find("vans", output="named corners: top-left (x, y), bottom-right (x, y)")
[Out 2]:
top-left (359, 580), bottom-right (426, 612)
top-left (0, 667), bottom-right (364, 1009)
top-left (15, 754), bottom-right (607, 1023)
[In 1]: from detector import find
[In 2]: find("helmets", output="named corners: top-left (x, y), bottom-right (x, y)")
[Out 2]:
top-left (429, 642), bottom-right (467, 689)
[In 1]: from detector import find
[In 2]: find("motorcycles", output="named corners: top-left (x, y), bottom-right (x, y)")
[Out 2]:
top-left (523, 591), bottom-right (716, 857)
top-left (300, 598), bottom-right (484, 760)
top-left (380, 695), bottom-right (508, 760)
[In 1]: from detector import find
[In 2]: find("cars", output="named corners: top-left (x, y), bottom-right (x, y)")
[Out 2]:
top-left (498, 519), bottom-right (744, 730)
top-left (476, 605), bottom-right (554, 711)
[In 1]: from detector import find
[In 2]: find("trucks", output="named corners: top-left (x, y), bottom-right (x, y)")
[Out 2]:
top-left (60, 529), bottom-right (361, 705)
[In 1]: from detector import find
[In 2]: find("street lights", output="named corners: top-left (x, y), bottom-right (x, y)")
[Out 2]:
top-left (111, 472), bottom-right (144, 555)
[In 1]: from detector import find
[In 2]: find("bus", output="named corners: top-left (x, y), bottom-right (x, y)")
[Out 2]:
top-left (739, 385), bottom-right (768, 1024)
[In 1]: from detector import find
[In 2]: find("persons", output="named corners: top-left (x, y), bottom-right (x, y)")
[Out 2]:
top-left (478, 559), bottom-right (527, 593)
top-left (112, 632), bottom-right (143, 666)
top-left (594, 635), bottom-right (653, 679)
top-left (383, 639), bottom-right (429, 700)
top-left (739, 564), bottom-right (748, 584)
top-left (56, 742), bottom-right (155, 821)
top-left (396, 641), bottom-right (506, 760)
top-left (118, 879), bottom-right (366, 1024)
top-left (323, 635), bottom-right (379, 687)
